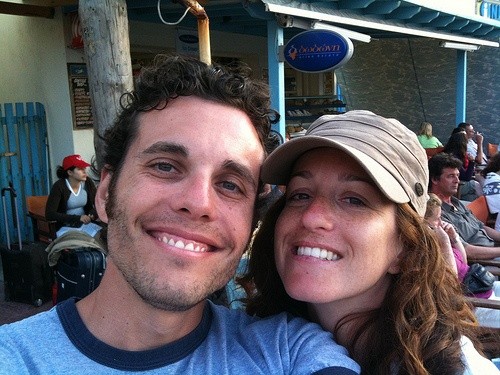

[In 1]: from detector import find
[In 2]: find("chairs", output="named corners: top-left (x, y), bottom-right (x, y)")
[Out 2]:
top-left (487, 143), bottom-right (498, 156)
top-left (463, 259), bottom-right (500, 309)
top-left (467, 196), bottom-right (495, 229)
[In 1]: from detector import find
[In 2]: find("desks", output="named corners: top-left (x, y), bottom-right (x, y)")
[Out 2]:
top-left (26, 211), bottom-right (58, 246)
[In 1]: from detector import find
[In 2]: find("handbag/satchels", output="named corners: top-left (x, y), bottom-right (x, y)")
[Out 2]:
top-left (461, 264), bottom-right (496, 295)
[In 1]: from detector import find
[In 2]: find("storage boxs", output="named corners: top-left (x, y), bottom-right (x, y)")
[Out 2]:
top-left (26, 196), bottom-right (49, 217)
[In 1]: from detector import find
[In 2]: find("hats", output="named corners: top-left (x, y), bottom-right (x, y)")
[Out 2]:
top-left (260, 110), bottom-right (430, 221)
top-left (62, 154), bottom-right (90, 171)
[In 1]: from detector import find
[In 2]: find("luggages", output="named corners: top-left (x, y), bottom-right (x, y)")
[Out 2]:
top-left (0, 187), bottom-right (54, 304)
top-left (57, 248), bottom-right (106, 299)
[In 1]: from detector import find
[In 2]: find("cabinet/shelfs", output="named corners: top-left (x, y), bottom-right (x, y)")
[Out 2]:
top-left (283, 96), bottom-right (346, 140)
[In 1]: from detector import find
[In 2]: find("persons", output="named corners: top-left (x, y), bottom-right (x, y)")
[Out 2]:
top-left (32, 154), bottom-right (107, 307)
top-left (0, 53), bottom-right (361, 375)
top-left (226, 110), bottom-right (500, 375)
top-left (428, 152), bottom-right (500, 281)
top-left (416, 121), bottom-right (444, 148)
top-left (424, 193), bottom-right (500, 328)
top-left (441, 122), bottom-right (500, 234)
